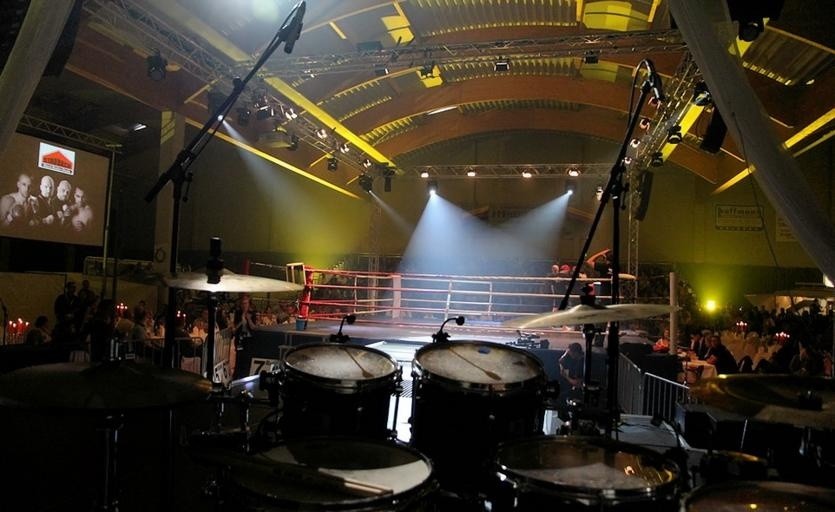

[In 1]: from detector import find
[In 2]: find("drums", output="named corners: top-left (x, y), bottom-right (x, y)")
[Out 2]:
top-left (408, 339), bottom-right (547, 501)
top-left (682, 478), bottom-right (835, 512)
top-left (201, 433), bottom-right (442, 512)
top-left (280, 341), bottom-right (398, 444)
top-left (487, 435), bottom-right (682, 510)
top-left (115, 414), bottom-right (249, 511)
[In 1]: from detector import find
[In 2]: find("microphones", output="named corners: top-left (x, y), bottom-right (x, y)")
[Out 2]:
top-left (644, 58), bottom-right (664, 100)
top-left (284, 1), bottom-right (305, 53)
top-left (329, 315), bottom-right (356, 343)
top-left (433, 316), bottom-right (464, 343)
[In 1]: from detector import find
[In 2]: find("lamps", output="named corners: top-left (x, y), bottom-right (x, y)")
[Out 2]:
top-left (373, 50), bottom-right (600, 78)
top-left (651, 77), bottom-right (713, 168)
top-left (208, 81), bottom-right (377, 196)
top-left (146, 46), bottom-right (171, 82)
top-left (428, 178), bottom-right (438, 197)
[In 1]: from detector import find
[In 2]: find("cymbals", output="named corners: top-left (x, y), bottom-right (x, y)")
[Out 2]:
top-left (0, 363), bottom-right (212, 410)
top-left (518, 304), bottom-right (683, 329)
top-left (157, 269), bottom-right (305, 294)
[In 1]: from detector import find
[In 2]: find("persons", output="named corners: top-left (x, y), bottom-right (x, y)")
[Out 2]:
top-left (0, 169), bottom-right (94, 238)
top-left (25, 281), bottom-right (297, 381)
top-left (656, 304), bottom-right (834, 374)
top-left (558, 342), bottom-right (584, 422)
top-left (547, 248), bottom-right (615, 346)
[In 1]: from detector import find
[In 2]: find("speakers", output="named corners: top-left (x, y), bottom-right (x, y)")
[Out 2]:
top-left (700, 106), bottom-right (727, 154)
top-left (634, 172), bottom-right (653, 221)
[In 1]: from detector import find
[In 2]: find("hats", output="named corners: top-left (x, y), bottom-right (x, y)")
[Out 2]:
top-left (666, 329), bottom-right (689, 349)
